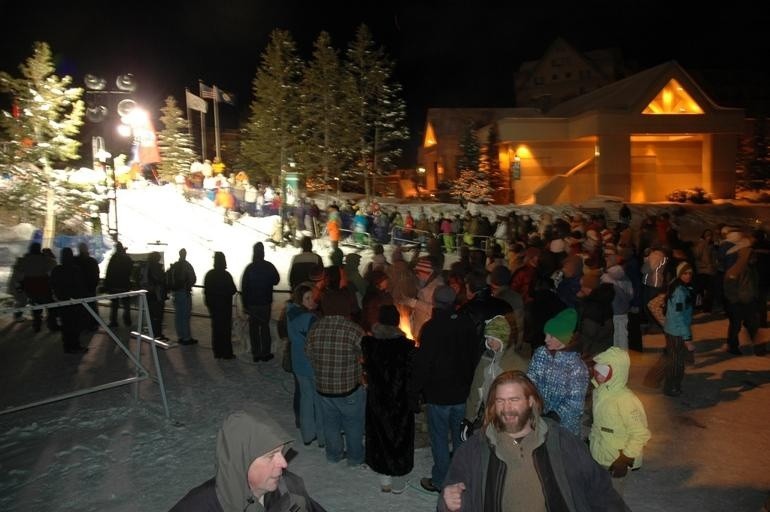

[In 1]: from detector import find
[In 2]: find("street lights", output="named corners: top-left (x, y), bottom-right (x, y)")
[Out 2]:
top-left (82, 73), bottom-right (138, 252)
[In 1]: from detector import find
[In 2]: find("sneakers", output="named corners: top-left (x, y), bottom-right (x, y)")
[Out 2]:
top-left (382, 479), bottom-right (441, 494)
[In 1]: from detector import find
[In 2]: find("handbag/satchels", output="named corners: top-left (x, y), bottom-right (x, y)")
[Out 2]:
top-left (164, 268), bottom-right (179, 292)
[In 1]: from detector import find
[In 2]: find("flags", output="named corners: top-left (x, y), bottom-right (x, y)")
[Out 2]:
top-left (186, 82), bottom-right (235, 114)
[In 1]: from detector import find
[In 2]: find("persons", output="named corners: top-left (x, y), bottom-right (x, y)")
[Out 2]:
top-left (170, 407), bottom-right (325, 511)
top-left (284, 153), bottom-right (770, 510)
top-left (9, 240), bottom-right (280, 361)
top-left (189, 153), bottom-right (281, 225)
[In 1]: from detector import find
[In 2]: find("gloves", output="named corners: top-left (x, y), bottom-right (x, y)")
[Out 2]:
top-left (459, 418), bottom-right (473, 443)
top-left (608, 450), bottom-right (634, 478)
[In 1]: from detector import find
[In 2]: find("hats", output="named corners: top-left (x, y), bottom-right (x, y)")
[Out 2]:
top-left (307, 241), bottom-right (693, 378)
